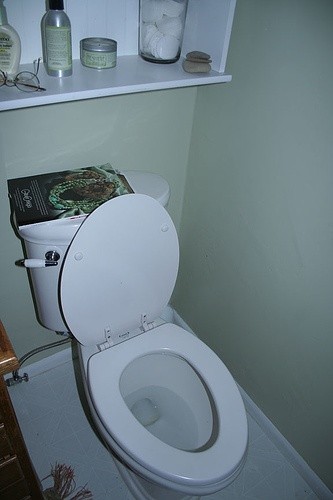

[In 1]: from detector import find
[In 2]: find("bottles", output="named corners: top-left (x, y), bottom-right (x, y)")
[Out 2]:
top-left (39, 1), bottom-right (72, 78)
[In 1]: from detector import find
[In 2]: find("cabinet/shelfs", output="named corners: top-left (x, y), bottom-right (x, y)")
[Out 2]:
top-left (1, 357), bottom-right (46, 500)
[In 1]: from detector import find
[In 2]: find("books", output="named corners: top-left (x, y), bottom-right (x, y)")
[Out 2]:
top-left (6, 162), bottom-right (129, 231)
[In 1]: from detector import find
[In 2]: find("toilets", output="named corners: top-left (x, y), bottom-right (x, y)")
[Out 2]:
top-left (11, 170), bottom-right (249, 499)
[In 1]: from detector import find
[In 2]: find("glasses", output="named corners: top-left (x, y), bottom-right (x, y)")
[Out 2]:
top-left (0, 58), bottom-right (46, 92)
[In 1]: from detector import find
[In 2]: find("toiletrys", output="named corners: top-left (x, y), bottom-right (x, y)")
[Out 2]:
top-left (0, 5), bottom-right (22, 79)
